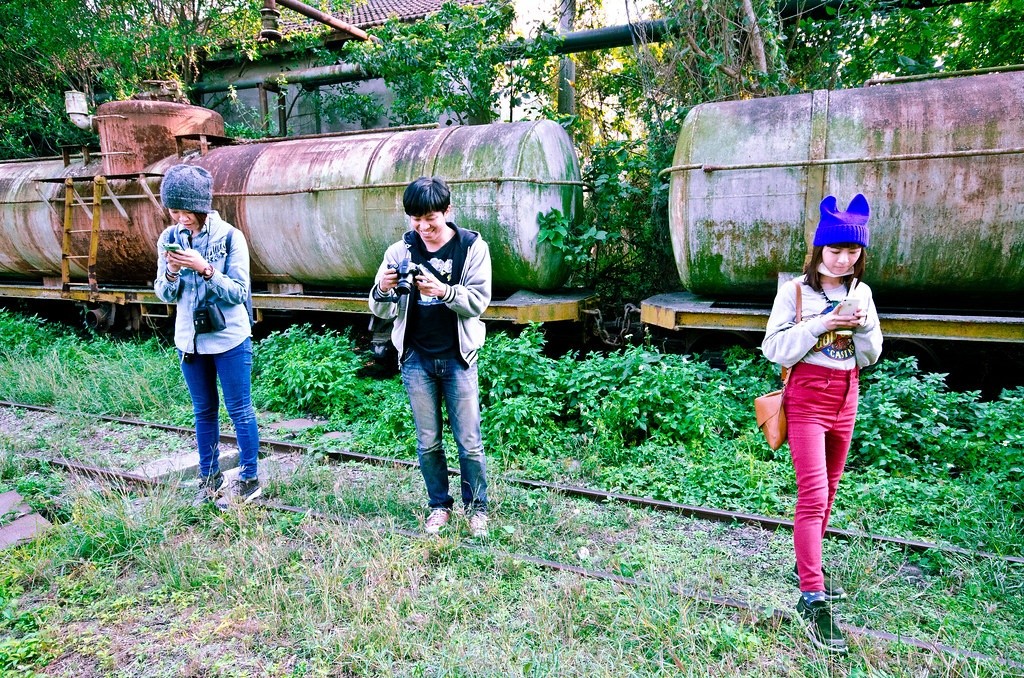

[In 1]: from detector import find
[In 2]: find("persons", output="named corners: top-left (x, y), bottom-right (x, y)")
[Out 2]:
top-left (368, 173), bottom-right (493, 540)
top-left (760, 191), bottom-right (882, 653)
top-left (153, 163), bottom-right (261, 504)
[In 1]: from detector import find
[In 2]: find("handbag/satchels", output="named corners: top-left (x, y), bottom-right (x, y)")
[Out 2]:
top-left (754, 390), bottom-right (787, 451)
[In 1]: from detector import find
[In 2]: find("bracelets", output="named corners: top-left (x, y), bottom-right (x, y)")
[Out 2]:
top-left (165, 269), bottom-right (180, 278)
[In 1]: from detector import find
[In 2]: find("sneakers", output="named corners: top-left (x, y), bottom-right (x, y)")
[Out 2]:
top-left (426, 509), bottom-right (451, 534)
top-left (216, 476), bottom-right (263, 511)
top-left (794, 595), bottom-right (848, 656)
top-left (793, 560), bottom-right (846, 600)
top-left (469, 510), bottom-right (489, 539)
top-left (193, 469), bottom-right (224, 508)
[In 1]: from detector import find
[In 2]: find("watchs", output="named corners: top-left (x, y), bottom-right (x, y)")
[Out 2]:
top-left (198, 265), bottom-right (213, 276)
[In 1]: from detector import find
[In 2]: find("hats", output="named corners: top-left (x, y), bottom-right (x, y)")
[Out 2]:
top-left (160, 163), bottom-right (214, 214)
top-left (812, 194), bottom-right (871, 247)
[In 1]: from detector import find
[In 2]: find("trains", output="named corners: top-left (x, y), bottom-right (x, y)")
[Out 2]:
top-left (0, 66), bottom-right (1024, 404)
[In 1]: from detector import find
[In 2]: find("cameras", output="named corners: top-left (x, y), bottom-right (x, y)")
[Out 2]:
top-left (387, 258), bottom-right (423, 296)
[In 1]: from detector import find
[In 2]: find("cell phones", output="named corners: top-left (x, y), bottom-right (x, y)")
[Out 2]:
top-left (162, 243), bottom-right (182, 255)
top-left (838, 298), bottom-right (860, 316)
